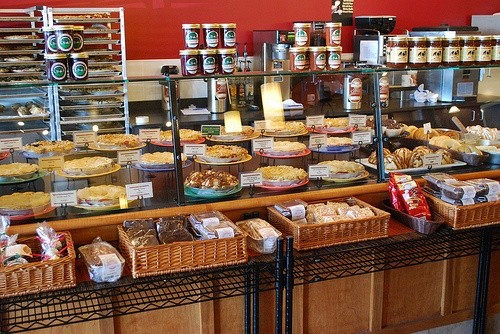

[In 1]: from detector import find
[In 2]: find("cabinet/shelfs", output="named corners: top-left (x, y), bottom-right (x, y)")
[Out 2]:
top-left (1, 7), bottom-right (128, 140)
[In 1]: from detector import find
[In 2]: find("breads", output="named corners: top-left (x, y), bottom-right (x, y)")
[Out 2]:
top-left (123, 216), bottom-right (193, 244)
top-left (291, 202), bottom-right (376, 224)
top-left (55, 13), bottom-right (120, 104)
top-left (82, 245), bottom-right (124, 267)
top-left (0, 35), bottom-right (43, 115)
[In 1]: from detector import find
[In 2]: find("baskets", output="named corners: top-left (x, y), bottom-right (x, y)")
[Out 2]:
top-left (381, 198), bottom-right (445, 236)
top-left (0, 231), bottom-right (76, 299)
top-left (266, 196), bottom-right (391, 251)
top-left (419, 186), bottom-right (500, 230)
top-left (116, 201), bottom-right (249, 278)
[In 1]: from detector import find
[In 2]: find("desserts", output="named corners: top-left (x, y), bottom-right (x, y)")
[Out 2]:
top-left (366, 120), bottom-right (500, 170)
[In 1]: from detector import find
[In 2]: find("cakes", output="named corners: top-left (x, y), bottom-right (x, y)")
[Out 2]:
top-left (0, 134), bottom-right (139, 213)
top-left (317, 116), bottom-right (366, 179)
top-left (140, 117), bottom-right (306, 189)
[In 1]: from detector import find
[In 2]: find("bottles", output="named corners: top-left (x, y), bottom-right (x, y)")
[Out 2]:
top-left (378, 73), bottom-right (389, 108)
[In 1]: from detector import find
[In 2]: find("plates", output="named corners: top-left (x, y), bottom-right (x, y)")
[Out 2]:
top-left (0, 126), bottom-right (466, 220)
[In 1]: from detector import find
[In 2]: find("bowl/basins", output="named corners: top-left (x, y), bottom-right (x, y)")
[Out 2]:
top-left (355, 15), bottom-right (396, 35)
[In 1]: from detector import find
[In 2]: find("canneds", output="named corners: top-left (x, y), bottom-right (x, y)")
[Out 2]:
top-left (386, 34), bottom-right (500, 70)
top-left (287, 21), bottom-right (342, 70)
top-left (179, 22), bottom-right (238, 75)
top-left (42, 25), bottom-right (89, 82)
